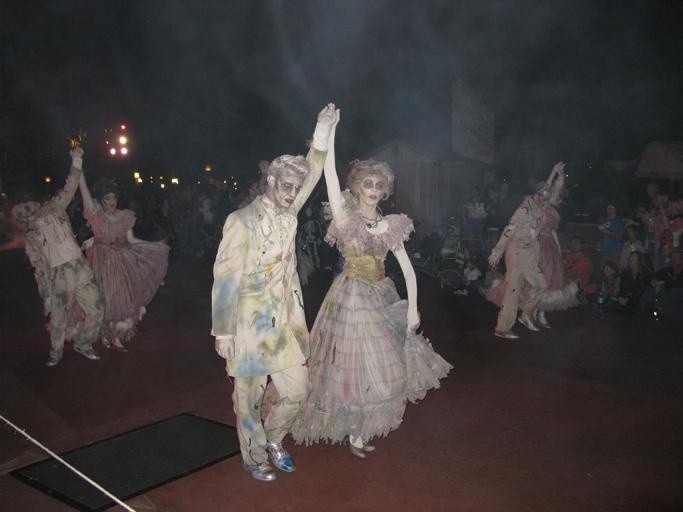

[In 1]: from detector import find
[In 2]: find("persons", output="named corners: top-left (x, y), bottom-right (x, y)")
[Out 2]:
top-left (208, 100), bottom-right (342, 483)
top-left (484, 160), bottom-right (564, 341)
top-left (55, 148), bottom-right (173, 356)
top-left (284, 104), bottom-right (459, 459)
top-left (513, 161), bottom-right (570, 334)
top-left (6, 142), bottom-right (104, 368)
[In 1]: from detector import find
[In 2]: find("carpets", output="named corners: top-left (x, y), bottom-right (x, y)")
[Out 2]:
top-left (7, 410), bottom-right (252, 510)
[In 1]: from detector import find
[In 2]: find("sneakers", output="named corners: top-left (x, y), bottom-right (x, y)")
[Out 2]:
top-left (493, 310), bottom-right (552, 340)
top-left (46, 336), bottom-right (128, 367)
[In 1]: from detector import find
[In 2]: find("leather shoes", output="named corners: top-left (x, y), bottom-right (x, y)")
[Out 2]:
top-left (241, 460), bottom-right (275, 481)
top-left (266, 441), bottom-right (297, 472)
top-left (362, 441), bottom-right (376, 452)
top-left (347, 434), bottom-right (367, 458)
